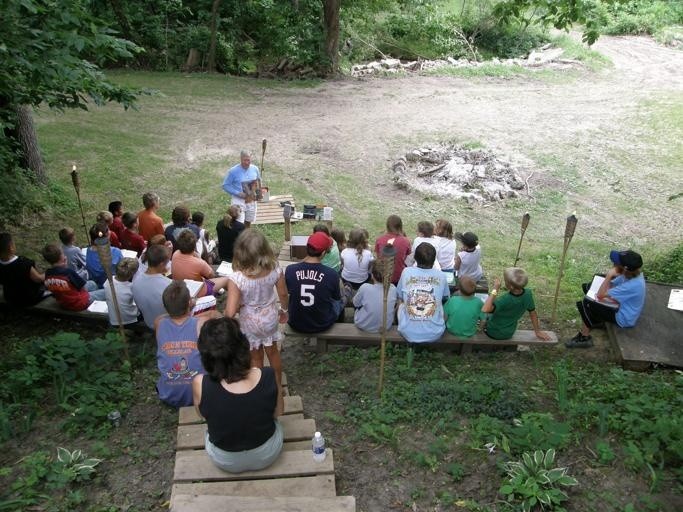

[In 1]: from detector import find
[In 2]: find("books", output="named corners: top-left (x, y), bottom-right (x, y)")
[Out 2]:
top-left (583, 274), bottom-right (619, 309)
top-left (121, 248), bottom-right (138, 259)
top-left (667, 287), bottom-right (683, 312)
top-left (87, 300), bottom-right (108, 313)
top-left (241, 179), bottom-right (263, 203)
top-left (216, 260), bottom-right (234, 278)
top-left (183, 278), bottom-right (205, 298)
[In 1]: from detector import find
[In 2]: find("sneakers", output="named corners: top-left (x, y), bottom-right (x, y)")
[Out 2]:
top-left (563, 331), bottom-right (595, 348)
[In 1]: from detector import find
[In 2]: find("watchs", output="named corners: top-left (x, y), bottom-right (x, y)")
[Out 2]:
top-left (491, 289), bottom-right (498, 296)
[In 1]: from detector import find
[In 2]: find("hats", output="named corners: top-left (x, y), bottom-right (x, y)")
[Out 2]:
top-left (609, 250), bottom-right (643, 272)
top-left (454, 231), bottom-right (479, 247)
top-left (307, 231), bottom-right (334, 252)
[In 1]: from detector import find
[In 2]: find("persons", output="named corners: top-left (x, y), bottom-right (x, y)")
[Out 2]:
top-left (37, 244), bottom-right (105, 313)
top-left (454, 230), bottom-right (484, 284)
top-left (214, 203), bottom-right (246, 261)
top-left (222, 149), bottom-right (264, 231)
top-left (280, 232), bottom-right (353, 335)
top-left (395, 241), bottom-right (452, 346)
top-left (102, 256), bottom-right (144, 328)
top-left (85, 222), bottom-right (124, 290)
top-left (563, 249), bottom-right (645, 347)
top-left (164, 239), bottom-right (174, 278)
top-left (0, 231), bottom-right (46, 310)
top-left (340, 227), bottom-right (377, 289)
top-left (349, 257), bottom-right (396, 336)
top-left (130, 233), bottom-right (166, 284)
top-left (152, 279), bottom-right (211, 407)
top-left (121, 210), bottom-right (147, 259)
top-left (138, 190), bottom-right (165, 242)
top-left (329, 229), bottom-right (347, 252)
top-left (432, 217), bottom-right (457, 274)
top-left (190, 210), bottom-right (218, 262)
top-left (312, 223), bottom-right (342, 277)
top-left (189, 315), bottom-right (287, 474)
top-left (164, 206), bottom-right (205, 258)
top-left (107, 200), bottom-right (125, 241)
top-left (371, 214), bottom-right (412, 282)
top-left (130, 241), bottom-right (217, 331)
top-left (58, 226), bottom-right (89, 283)
top-left (401, 220), bottom-right (442, 270)
top-left (479, 266), bottom-right (553, 342)
top-left (223, 225), bottom-right (291, 420)
top-left (443, 275), bottom-right (488, 340)
top-left (170, 229), bottom-right (228, 298)
top-left (95, 210), bottom-right (123, 248)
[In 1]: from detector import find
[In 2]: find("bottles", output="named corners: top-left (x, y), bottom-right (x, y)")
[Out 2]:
top-left (313, 431), bottom-right (326, 462)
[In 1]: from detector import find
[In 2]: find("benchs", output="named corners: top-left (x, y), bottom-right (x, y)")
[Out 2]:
top-left (283, 321), bottom-right (558, 356)
top-left (32, 295), bottom-right (109, 322)
